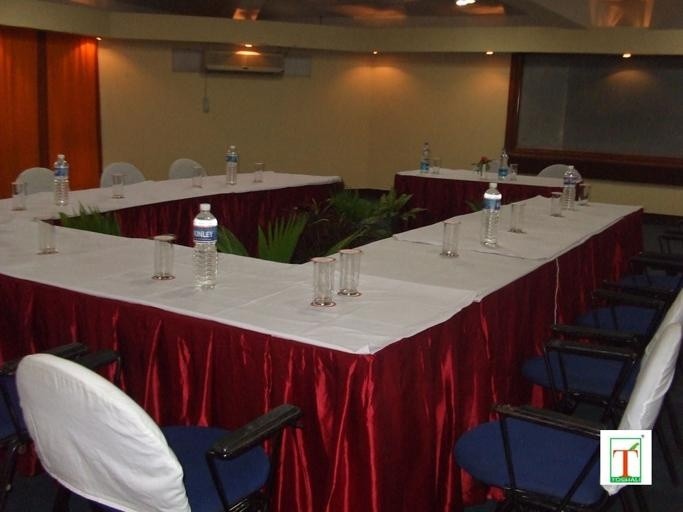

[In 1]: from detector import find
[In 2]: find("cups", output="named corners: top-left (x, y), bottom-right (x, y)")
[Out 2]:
top-left (548, 191), bottom-right (563, 215)
top-left (310, 256), bottom-right (337, 307)
top-left (191, 165), bottom-right (204, 186)
top-left (109, 173), bottom-right (125, 198)
top-left (152, 236), bottom-right (174, 279)
top-left (10, 181), bottom-right (28, 213)
top-left (504, 163), bottom-right (521, 182)
top-left (35, 214), bottom-right (57, 254)
top-left (509, 202), bottom-right (527, 232)
top-left (577, 181), bottom-right (594, 206)
top-left (251, 161), bottom-right (264, 181)
top-left (429, 156), bottom-right (442, 174)
top-left (440, 217), bottom-right (460, 256)
top-left (338, 250), bottom-right (361, 298)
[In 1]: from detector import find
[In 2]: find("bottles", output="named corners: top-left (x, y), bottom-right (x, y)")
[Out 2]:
top-left (420, 137), bottom-right (431, 174)
top-left (561, 164), bottom-right (575, 211)
top-left (52, 153), bottom-right (70, 205)
top-left (191, 202), bottom-right (218, 288)
top-left (481, 181), bottom-right (502, 249)
top-left (497, 148), bottom-right (507, 179)
top-left (225, 145), bottom-right (239, 183)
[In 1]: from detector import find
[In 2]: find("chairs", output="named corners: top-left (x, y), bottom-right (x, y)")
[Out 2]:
top-left (12, 161), bottom-right (66, 196)
top-left (99, 157), bottom-right (143, 186)
top-left (451, 225), bottom-right (681, 511)
top-left (472, 158), bottom-right (513, 175)
top-left (164, 157), bottom-right (204, 178)
top-left (13, 345), bottom-right (301, 510)
top-left (0, 310), bottom-right (83, 469)
top-left (535, 162), bottom-right (578, 183)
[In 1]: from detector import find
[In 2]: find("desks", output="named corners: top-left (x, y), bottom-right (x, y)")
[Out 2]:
top-left (391, 164), bottom-right (587, 225)
top-left (0, 222), bottom-right (370, 512)
top-left (0, 167), bottom-right (343, 251)
top-left (372, 195), bottom-right (644, 510)
